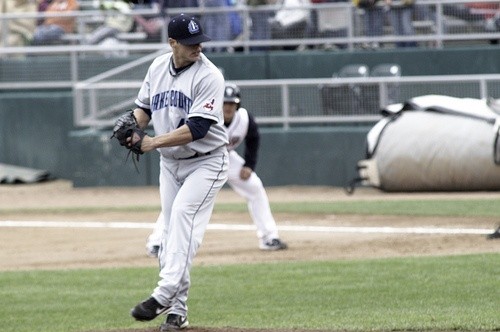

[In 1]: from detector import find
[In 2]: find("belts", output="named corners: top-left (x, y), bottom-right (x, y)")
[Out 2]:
top-left (178, 150), bottom-right (211, 160)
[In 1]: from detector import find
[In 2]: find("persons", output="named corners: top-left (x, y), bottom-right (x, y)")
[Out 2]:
top-left (114, 13), bottom-right (229, 329)
top-left (31, 0), bottom-right (78, 44)
top-left (148, 82), bottom-right (289, 255)
top-left (123, 0), bottom-right (500, 53)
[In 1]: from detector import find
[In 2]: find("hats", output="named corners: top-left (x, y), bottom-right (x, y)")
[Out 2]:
top-left (168, 13), bottom-right (211, 45)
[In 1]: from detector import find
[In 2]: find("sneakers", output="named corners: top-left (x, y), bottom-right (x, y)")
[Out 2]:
top-left (160, 314), bottom-right (190, 330)
top-left (131, 297), bottom-right (172, 322)
top-left (259, 238), bottom-right (287, 250)
top-left (145, 244), bottom-right (159, 258)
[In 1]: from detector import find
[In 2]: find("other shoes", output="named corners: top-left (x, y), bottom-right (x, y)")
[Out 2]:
top-left (488, 226), bottom-right (500, 239)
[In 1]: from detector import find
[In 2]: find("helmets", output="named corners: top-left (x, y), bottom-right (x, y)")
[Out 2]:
top-left (224, 83), bottom-right (241, 104)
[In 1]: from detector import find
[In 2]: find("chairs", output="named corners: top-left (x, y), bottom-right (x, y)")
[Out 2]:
top-left (319, 63), bottom-right (401, 114)
